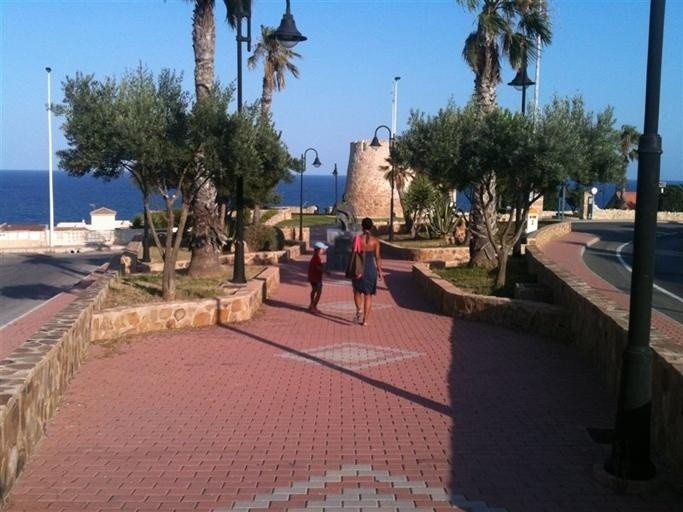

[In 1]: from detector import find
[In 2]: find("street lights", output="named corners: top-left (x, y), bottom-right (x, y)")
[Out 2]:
top-left (588, 186), bottom-right (597, 220)
top-left (369, 123), bottom-right (395, 243)
top-left (232, 0), bottom-right (308, 283)
top-left (298, 147), bottom-right (322, 243)
top-left (44, 66), bottom-right (56, 254)
top-left (332, 164), bottom-right (339, 203)
top-left (390, 75), bottom-right (399, 139)
top-left (506, 45), bottom-right (536, 256)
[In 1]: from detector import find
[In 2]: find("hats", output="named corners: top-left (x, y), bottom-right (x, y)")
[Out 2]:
top-left (312, 241), bottom-right (329, 250)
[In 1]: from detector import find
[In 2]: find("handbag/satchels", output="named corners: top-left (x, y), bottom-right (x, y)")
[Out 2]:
top-left (344, 250), bottom-right (365, 281)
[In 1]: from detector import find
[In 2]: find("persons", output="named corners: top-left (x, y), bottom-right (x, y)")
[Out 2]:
top-left (351, 217), bottom-right (384, 328)
top-left (307, 241), bottom-right (331, 314)
